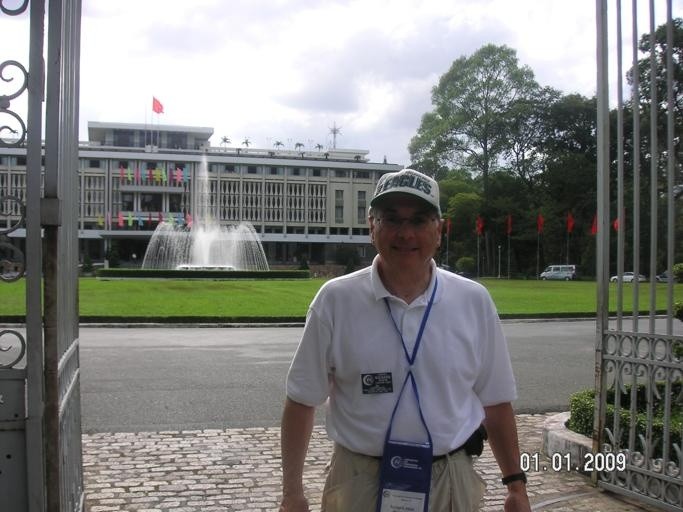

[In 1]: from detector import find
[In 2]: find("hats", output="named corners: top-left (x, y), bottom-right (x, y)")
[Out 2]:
top-left (370, 169), bottom-right (440, 211)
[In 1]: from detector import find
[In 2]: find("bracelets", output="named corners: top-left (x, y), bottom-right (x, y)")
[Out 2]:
top-left (500, 470), bottom-right (527, 485)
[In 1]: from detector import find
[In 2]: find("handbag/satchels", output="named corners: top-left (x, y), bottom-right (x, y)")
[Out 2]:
top-left (465, 423), bottom-right (488, 457)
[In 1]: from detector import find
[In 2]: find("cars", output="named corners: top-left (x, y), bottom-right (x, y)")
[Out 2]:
top-left (610, 272), bottom-right (647, 283)
top-left (656, 275), bottom-right (668, 283)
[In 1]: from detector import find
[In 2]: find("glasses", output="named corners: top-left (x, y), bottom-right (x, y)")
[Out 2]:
top-left (375, 214), bottom-right (436, 229)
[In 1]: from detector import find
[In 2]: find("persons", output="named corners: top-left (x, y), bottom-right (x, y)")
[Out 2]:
top-left (278, 167), bottom-right (531, 512)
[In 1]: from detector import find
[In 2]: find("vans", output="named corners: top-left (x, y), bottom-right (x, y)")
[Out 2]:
top-left (540, 265), bottom-right (576, 281)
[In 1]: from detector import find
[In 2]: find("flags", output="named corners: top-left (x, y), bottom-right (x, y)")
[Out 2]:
top-left (95, 208), bottom-right (192, 229)
top-left (506, 213), bottom-right (512, 233)
top-left (151, 96), bottom-right (164, 114)
top-left (566, 209), bottom-right (574, 233)
top-left (613, 218), bottom-right (618, 231)
top-left (592, 211), bottom-right (596, 233)
top-left (475, 214), bottom-right (482, 234)
top-left (536, 209), bottom-right (544, 235)
top-left (118, 164), bottom-right (188, 184)
top-left (446, 217), bottom-right (451, 234)
